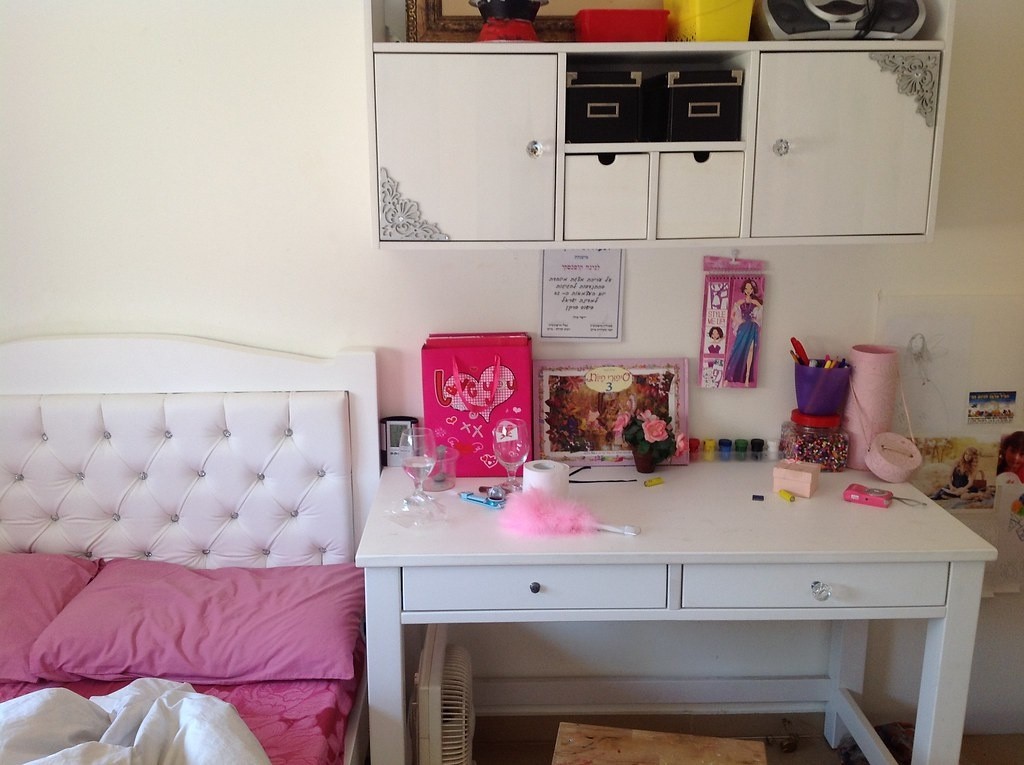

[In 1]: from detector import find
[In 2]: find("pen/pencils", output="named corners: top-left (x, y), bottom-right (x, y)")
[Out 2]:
top-left (840, 358), bottom-right (846, 368)
top-left (825, 354), bottom-right (831, 361)
top-left (804, 361), bottom-right (831, 412)
top-left (830, 356), bottom-right (838, 368)
top-left (791, 337), bottom-right (809, 366)
top-left (790, 350), bottom-right (800, 365)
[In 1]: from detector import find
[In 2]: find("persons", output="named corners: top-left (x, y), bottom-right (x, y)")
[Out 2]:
top-left (996, 431), bottom-right (1024, 483)
top-left (942, 447), bottom-right (991, 500)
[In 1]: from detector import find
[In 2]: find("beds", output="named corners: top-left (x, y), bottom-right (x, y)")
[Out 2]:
top-left (0, 332), bottom-right (381, 765)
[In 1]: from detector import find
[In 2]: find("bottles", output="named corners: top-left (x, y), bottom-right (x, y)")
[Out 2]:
top-left (779, 409), bottom-right (850, 473)
top-left (840, 345), bottom-right (899, 470)
top-left (689, 438), bottom-right (778, 462)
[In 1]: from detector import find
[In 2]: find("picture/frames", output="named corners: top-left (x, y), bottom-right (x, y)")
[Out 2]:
top-left (533, 358), bottom-right (690, 466)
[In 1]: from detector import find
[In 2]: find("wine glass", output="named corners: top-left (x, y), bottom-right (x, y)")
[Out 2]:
top-left (493, 417), bottom-right (530, 493)
top-left (398, 427), bottom-right (437, 510)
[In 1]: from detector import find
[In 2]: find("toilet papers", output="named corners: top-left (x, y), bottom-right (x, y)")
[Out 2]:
top-left (521, 459), bottom-right (569, 504)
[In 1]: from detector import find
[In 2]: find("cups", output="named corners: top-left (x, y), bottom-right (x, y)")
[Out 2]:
top-left (795, 359), bottom-right (853, 417)
top-left (422, 445), bottom-right (459, 492)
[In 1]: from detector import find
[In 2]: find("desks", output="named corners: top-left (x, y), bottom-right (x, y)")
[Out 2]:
top-left (355, 461), bottom-right (999, 765)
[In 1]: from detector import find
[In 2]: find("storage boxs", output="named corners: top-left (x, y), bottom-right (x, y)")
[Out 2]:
top-left (574, 9), bottom-right (671, 42)
top-left (773, 459), bottom-right (822, 498)
top-left (642, 70), bottom-right (744, 142)
top-left (565, 71), bottom-right (644, 142)
top-left (663, 0), bottom-right (755, 42)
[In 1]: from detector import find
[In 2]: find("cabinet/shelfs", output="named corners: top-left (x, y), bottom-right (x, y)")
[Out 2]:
top-left (363, 0), bottom-right (954, 247)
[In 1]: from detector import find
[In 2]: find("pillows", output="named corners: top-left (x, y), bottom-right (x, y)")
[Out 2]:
top-left (30, 558), bottom-right (364, 683)
top-left (0, 553), bottom-right (106, 682)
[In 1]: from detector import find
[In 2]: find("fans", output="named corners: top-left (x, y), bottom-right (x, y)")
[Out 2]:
top-left (407, 624), bottom-right (476, 765)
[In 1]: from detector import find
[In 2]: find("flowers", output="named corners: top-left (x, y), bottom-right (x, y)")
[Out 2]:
top-left (612, 407), bottom-right (687, 462)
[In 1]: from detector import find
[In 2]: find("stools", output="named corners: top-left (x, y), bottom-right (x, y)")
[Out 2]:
top-left (550, 721), bottom-right (768, 765)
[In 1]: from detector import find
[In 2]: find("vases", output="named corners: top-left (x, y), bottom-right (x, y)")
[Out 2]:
top-left (633, 447), bottom-right (657, 472)
